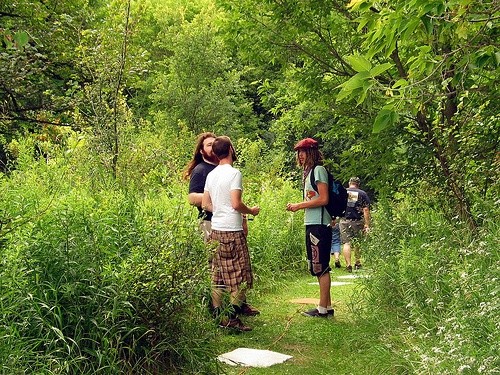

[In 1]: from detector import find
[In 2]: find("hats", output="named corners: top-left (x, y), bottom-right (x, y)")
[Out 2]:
top-left (293, 137), bottom-right (318, 148)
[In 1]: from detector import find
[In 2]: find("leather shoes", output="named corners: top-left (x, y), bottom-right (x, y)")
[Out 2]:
top-left (219, 316), bottom-right (252, 333)
top-left (236, 302), bottom-right (259, 315)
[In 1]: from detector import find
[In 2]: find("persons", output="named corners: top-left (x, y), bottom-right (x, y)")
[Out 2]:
top-left (285, 137), bottom-right (334, 318)
top-left (340, 177), bottom-right (371, 271)
top-left (330, 216), bottom-right (341, 269)
top-left (183, 132), bottom-right (259, 318)
top-left (201, 136), bottom-right (260, 331)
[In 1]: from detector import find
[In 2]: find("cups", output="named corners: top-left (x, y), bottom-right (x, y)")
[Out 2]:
top-left (247, 213), bottom-right (255, 222)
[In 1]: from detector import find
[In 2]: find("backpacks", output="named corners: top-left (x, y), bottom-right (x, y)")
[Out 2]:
top-left (310, 163), bottom-right (348, 220)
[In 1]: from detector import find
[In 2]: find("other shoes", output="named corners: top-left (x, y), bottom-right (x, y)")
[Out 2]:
top-left (335, 259), bottom-right (341, 267)
top-left (300, 307), bottom-right (334, 318)
top-left (209, 307), bottom-right (234, 318)
top-left (346, 265), bottom-right (352, 272)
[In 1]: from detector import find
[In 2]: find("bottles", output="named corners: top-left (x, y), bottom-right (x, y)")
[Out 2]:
top-left (306, 190), bottom-right (318, 200)
top-left (241, 215), bottom-right (248, 238)
top-left (331, 216), bottom-right (336, 229)
